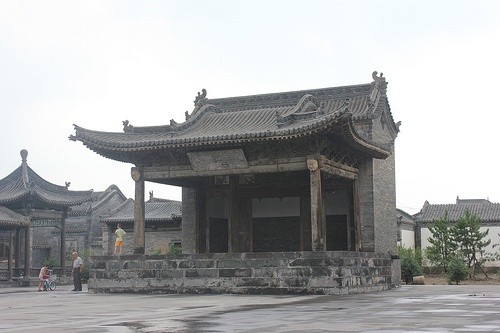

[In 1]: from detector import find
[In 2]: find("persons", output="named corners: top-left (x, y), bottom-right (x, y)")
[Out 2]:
top-left (71, 251), bottom-right (83, 292)
top-left (113, 223), bottom-right (126, 255)
top-left (38, 264), bottom-right (50, 292)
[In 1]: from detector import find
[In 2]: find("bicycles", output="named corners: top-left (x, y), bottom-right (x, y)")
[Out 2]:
top-left (44, 269), bottom-right (56, 292)
